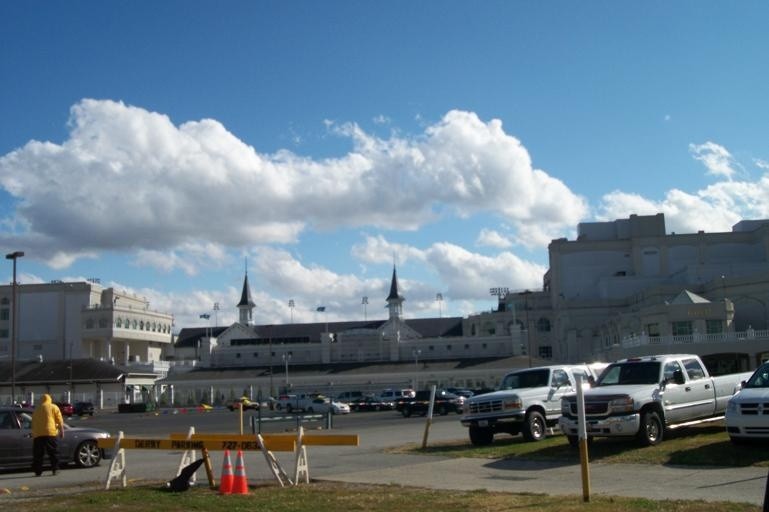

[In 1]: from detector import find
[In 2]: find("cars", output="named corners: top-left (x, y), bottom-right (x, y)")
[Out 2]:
top-left (348, 395), bottom-right (396, 412)
top-left (0, 407), bottom-right (113, 472)
top-left (304, 397), bottom-right (351, 415)
top-left (227, 397), bottom-right (260, 412)
top-left (397, 388), bottom-right (472, 416)
top-left (56, 402), bottom-right (73, 416)
top-left (75, 402), bottom-right (94, 416)
top-left (726, 362), bottom-right (769, 444)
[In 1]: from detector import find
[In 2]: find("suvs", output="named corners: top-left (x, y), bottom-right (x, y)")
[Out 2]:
top-left (460, 361), bottom-right (612, 446)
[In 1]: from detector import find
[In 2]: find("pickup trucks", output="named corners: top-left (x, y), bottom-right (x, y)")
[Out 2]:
top-left (559, 354), bottom-right (754, 445)
top-left (273, 394), bottom-right (323, 412)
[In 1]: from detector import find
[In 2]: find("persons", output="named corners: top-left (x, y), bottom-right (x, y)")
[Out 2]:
top-left (32, 393), bottom-right (65, 476)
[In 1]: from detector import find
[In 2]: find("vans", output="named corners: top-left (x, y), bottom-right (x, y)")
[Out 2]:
top-left (379, 388), bottom-right (416, 402)
top-left (335, 391), bottom-right (365, 402)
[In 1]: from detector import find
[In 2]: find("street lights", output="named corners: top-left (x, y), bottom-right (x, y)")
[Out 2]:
top-left (5, 251), bottom-right (25, 402)
top-left (412, 350), bottom-right (422, 392)
top-left (281, 354), bottom-right (291, 393)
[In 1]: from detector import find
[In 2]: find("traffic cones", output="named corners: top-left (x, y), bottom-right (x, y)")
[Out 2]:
top-left (231, 450), bottom-right (249, 494)
top-left (219, 450), bottom-right (235, 494)
